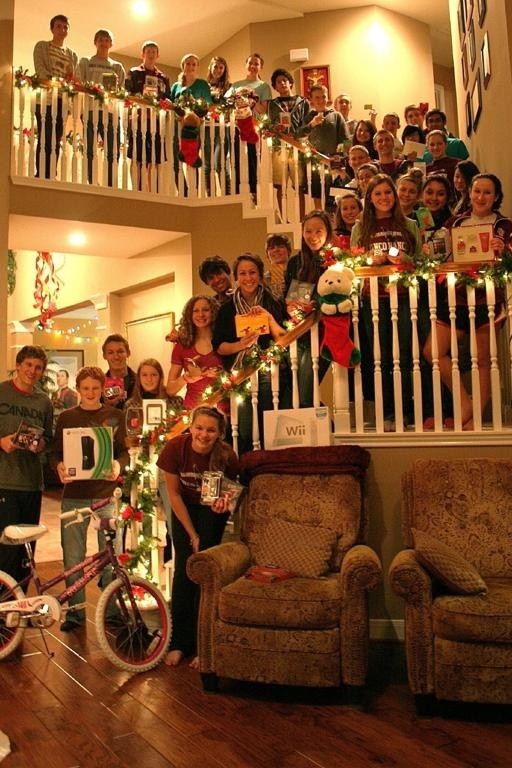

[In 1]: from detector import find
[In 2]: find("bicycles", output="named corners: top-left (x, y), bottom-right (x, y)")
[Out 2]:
top-left (1, 483), bottom-right (176, 678)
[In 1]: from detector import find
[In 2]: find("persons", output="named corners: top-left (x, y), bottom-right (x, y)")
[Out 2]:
top-left (76, 29), bottom-right (125, 187)
top-left (266, 68), bottom-right (310, 223)
top-left (122, 358), bottom-right (184, 565)
top-left (167, 234), bottom-right (292, 452)
top-left (1, 346), bottom-right (47, 602)
top-left (33, 16), bottom-right (78, 179)
top-left (298, 84), bottom-right (349, 210)
top-left (124, 41), bottom-right (172, 194)
top-left (282, 103), bottom-right (511, 431)
top-left (100, 334), bottom-right (137, 410)
top-left (157, 408), bottom-right (245, 670)
top-left (51, 370), bottom-right (78, 409)
top-left (201, 57), bottom-right (233, 197)
top-left (52, 367), bottom-right (127, 631)
top-left (170, 54), bottom-right (212, 197)
top-left (224, 53), bottom-right (272, 205)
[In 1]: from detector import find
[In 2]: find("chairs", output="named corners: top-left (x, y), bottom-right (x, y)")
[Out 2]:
top-left (387, 457), bottom-right (512, 719)
top-left (185, 474), bottom-right (383, 701)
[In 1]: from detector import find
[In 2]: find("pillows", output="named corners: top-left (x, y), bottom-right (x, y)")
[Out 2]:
top-left (255, 519), bottom-right (338, 579)
top-left (410, 528), bottom-right (489, 595)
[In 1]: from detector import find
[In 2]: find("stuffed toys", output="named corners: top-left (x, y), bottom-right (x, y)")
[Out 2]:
top-left (234, 95), bottom-right (259, 143)
top-left (177, 111), bottom-right (202, 167)
top-left (317, 267), bottom-right (362, 369)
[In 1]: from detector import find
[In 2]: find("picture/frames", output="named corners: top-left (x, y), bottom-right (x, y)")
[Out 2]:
top-left (450, 222), bottom-right (496, 262)
top-left (43, 350), bottom-right (84, 438)
top-left (300, 64), bottom-right (333, 104)
top-left (456, 0), bottom-right (491, 137)
